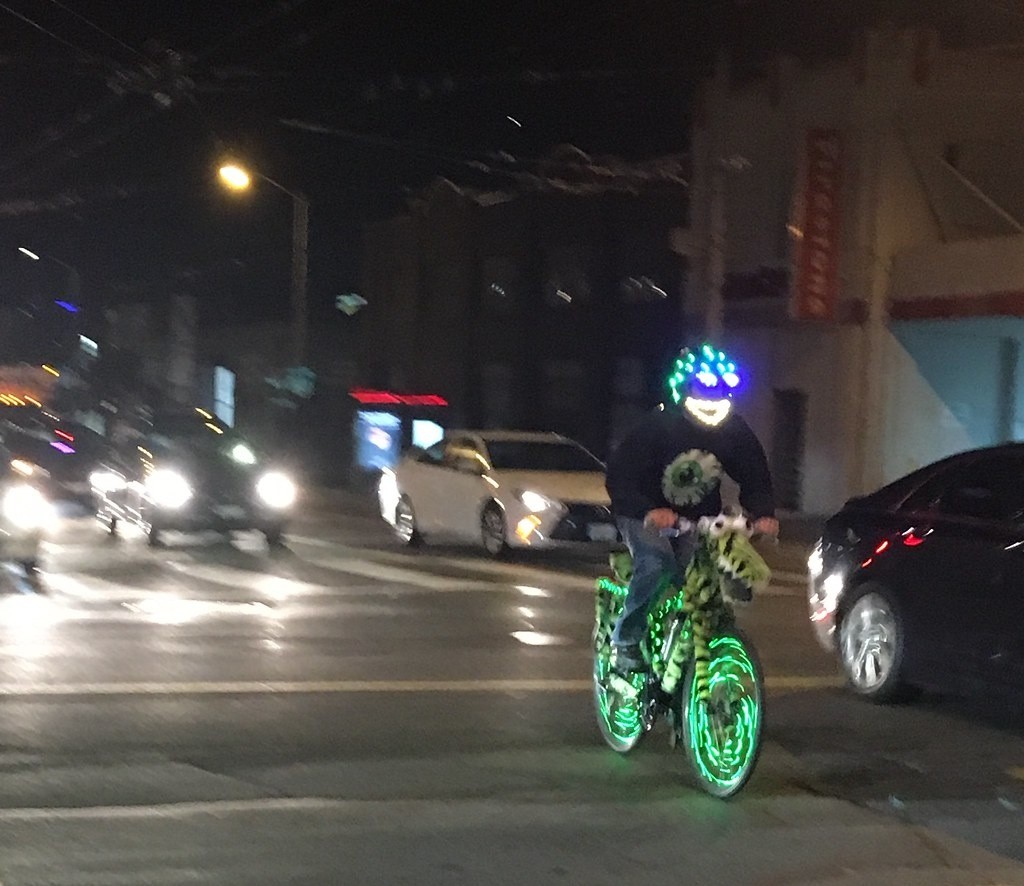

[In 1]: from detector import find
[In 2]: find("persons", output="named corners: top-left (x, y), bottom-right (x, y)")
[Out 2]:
top-left (604, 343), bottom-right (781, 747)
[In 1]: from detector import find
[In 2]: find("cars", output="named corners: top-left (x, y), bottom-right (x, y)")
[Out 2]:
top-left (0, 444), bottom-right (49, 574)
top-left (378, 430), bottom-right (629, 563)
top-left (806, 441), bottom-right (1024, 706)
top-left (87, 406), bottom-right (299, 549)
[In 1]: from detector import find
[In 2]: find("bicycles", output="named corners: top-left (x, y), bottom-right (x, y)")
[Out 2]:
top-left (591, 515), bottom-right (784, 799)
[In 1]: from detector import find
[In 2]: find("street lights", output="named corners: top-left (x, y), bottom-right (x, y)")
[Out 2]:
top-left (220, 165), bottom-right (311, 358)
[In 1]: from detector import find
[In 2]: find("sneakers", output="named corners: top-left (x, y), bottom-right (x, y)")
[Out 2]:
top-left (616, 645), bottom-right (650, 673)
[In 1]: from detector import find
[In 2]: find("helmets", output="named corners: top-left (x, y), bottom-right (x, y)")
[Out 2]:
top-left (667, 344), bottom-right (741, 406)
